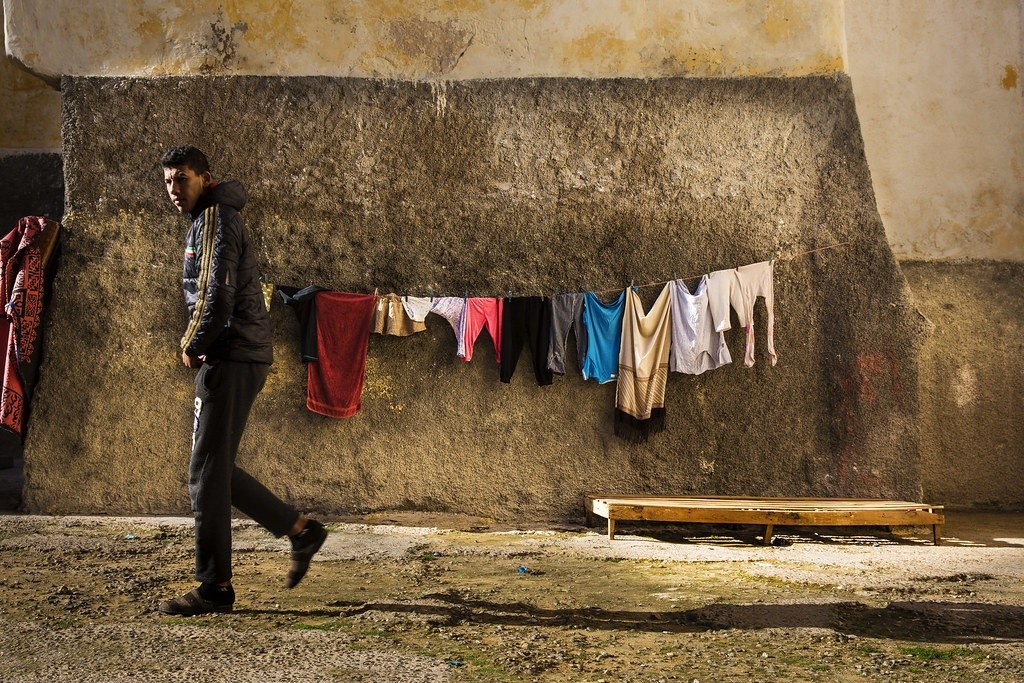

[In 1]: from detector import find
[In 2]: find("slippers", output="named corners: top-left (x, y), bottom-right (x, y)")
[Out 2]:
top-left (159, 589), bottom-right (233, 615)
top-left (286, 527), bottom-right (329, 589)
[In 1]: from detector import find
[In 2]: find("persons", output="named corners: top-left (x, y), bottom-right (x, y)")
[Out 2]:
top-left (157, 145), bottom-right (328, 616)
